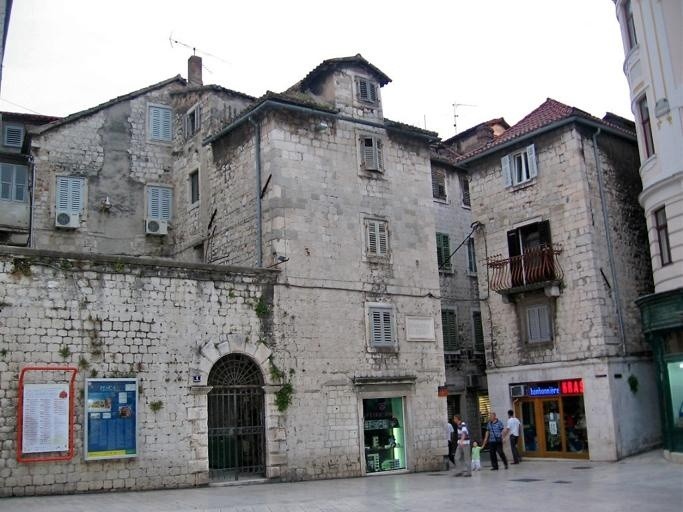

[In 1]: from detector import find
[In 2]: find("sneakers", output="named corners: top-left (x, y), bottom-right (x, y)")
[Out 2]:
top-left (455, 473), bottom-right (472, 477)
top-left (510, 460), bottom-right (522, 464)
top-left (472, 468), bottom-right (481, 471)
top-left (505, 466), bottom-right (508, 469)
top-left (491, 468), bottom-right (499, 470)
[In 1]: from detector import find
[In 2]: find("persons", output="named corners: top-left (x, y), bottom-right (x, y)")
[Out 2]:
top-left (482, 413), bottom-right (507, 470)
top-left (502, 409), bottom-right (522, 463)
top-left (453, 414), bottom-right (472, 477)
top-left (443, 422), bottom-right (454, 464)
top-left (471, 440), bottom-right (485, 471)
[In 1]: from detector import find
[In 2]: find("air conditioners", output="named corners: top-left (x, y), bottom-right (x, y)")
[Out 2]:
top-left (54, 209), bottom-right (80, 229)
top-left (144, 218), bottom-right (167, 236)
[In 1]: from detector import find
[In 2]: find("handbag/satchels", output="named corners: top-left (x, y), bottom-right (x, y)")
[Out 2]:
top-left (495, 437), bottom-right (503, 445)
